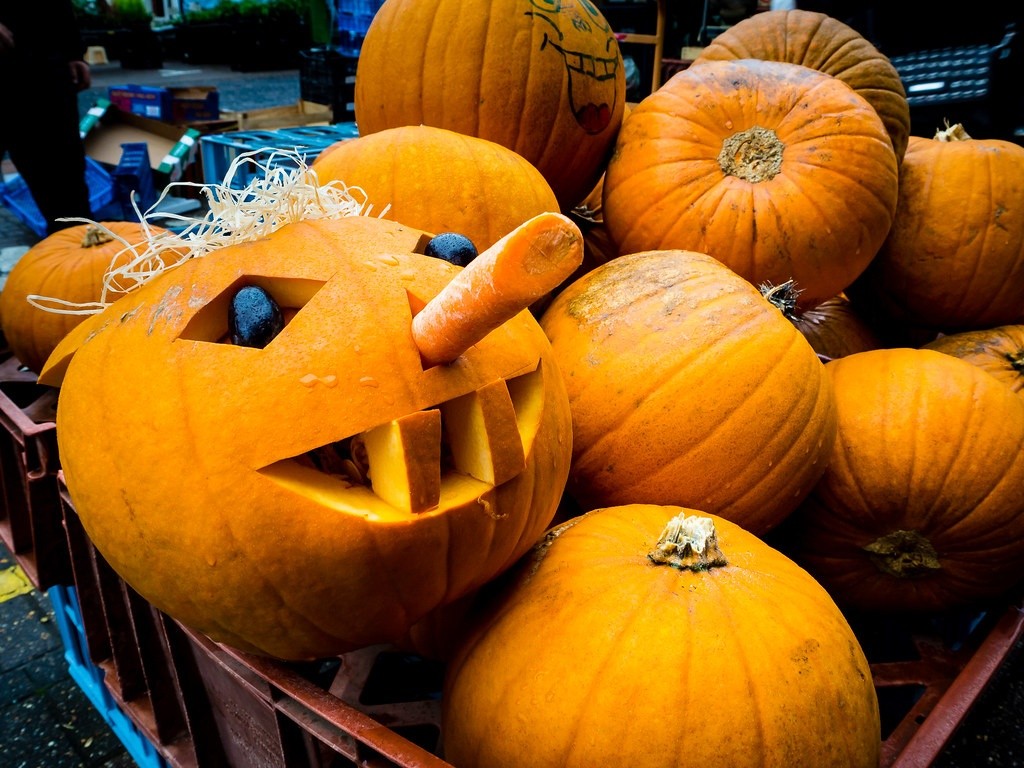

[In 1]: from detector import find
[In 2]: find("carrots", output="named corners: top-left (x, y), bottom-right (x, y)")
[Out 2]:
top-left (412, 211), bottom-right (584, 366)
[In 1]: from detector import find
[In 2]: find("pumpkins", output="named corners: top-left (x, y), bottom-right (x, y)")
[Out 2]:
top-left (0, 0), bottom-right (1024, 768)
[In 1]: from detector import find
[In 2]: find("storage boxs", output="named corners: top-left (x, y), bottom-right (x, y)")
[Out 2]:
top-left (0, 0), bottom-right (1024, 768)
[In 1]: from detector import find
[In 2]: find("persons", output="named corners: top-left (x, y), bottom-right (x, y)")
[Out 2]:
top-left (0, 0), bottom-right (111, 229)
